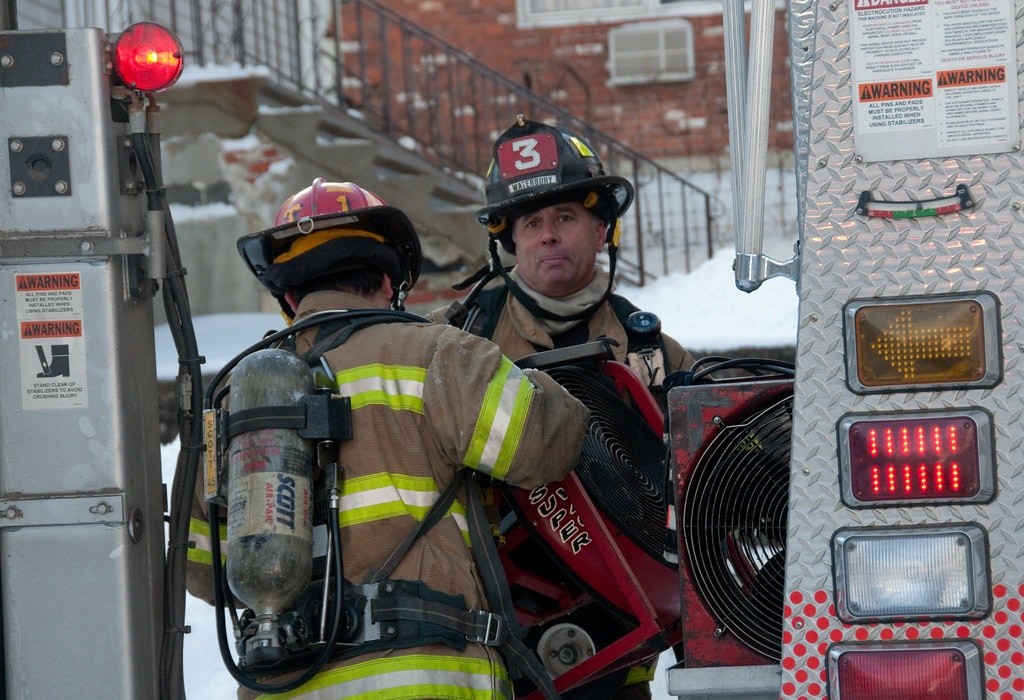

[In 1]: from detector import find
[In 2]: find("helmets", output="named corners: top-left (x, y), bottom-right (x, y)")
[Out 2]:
top-left (236, 177), bottom-right (422, 327)
top-left (452, 114), bottom-right (635, 248)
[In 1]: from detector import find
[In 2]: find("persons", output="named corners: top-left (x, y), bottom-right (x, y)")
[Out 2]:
top-left (421, 115), bottom-right (717, 700)
top-left (184, 176), bottom-right (592, 700)
